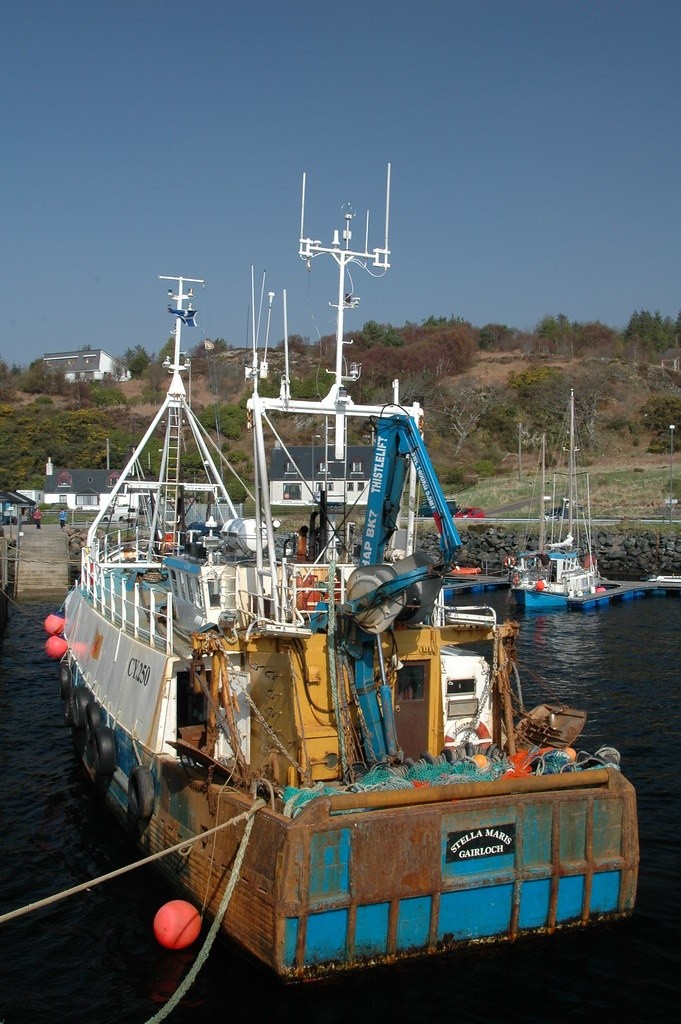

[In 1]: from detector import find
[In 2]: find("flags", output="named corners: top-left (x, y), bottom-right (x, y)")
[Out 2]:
top-left (169, 309), bottom-right (198, 327)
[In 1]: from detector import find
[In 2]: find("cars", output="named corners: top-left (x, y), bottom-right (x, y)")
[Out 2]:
top-left (539, 507), bottom-right (587, 520)
top-left (452, 508), bottom-right (485, 518)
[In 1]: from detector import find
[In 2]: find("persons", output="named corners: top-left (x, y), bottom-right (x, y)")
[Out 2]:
top-left (58, 508), bottom-right (68, 528)
top-left (34, 509), bottom-right (42, 529)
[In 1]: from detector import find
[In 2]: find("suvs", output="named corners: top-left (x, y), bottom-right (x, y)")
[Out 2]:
top-left (102, 507), bottom-right (137, 522)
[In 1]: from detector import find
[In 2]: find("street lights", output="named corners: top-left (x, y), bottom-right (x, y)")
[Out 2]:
top-left (7, 504), bottom-right (20, 599)
top-left (669, 425), bottom-right (676, 525)
top-left (193, 460), bottom-right (209, 521)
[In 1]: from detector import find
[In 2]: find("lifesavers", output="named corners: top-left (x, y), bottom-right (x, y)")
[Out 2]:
top-left (504, 556), bottom-right (516, 567)
top-left (452, 565), bottom-right (482, 574)
top-left (288, 574), bottom-right (305, 611)
top-left (445, 716), bottom-right (491, 760)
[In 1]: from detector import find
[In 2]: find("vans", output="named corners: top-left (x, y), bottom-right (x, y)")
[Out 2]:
top-left (414, 498), bottom-right (457, 517)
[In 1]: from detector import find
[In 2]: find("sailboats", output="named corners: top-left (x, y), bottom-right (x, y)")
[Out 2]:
top-left (510, 388), bottom-right (602, 609)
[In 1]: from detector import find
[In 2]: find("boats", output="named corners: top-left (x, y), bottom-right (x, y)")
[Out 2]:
top-left (647, 574), bottom-right (681, 582)
top-left (45, 164), bottom-right (640, 982)
